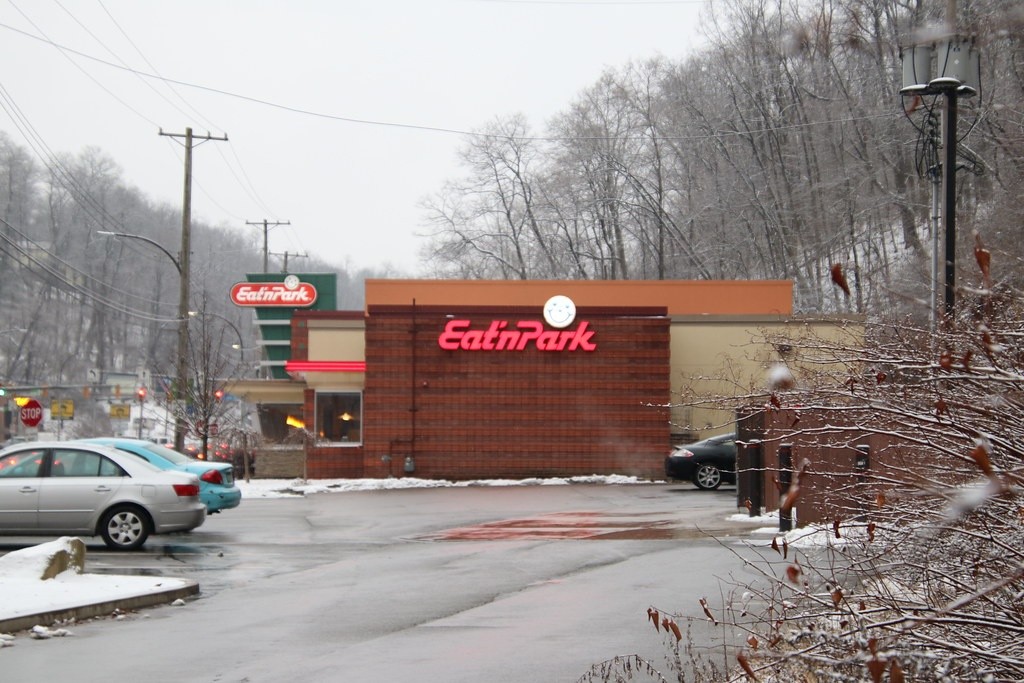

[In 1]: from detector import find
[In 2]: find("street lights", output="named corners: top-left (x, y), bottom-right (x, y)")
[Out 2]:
top-left (203, 308), bottom-right (247, 364)
top-left (98, 225), bottom-right (182, 280)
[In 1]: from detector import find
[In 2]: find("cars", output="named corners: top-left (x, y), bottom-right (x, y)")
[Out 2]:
top-left (76, 437), bottom-right (243, 515)
top-left (662, 431), bottom-right (874, 491)
top-left (1, 441), bottom-right (206, 549)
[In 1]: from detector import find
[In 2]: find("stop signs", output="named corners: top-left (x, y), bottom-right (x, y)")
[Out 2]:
top-left (20, 399), bottom-right (43, 427)
